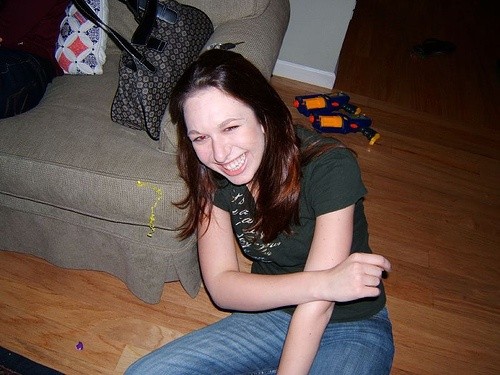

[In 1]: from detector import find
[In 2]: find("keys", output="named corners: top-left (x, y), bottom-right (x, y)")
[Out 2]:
top-left (220, 41), bottom-right (246, 49)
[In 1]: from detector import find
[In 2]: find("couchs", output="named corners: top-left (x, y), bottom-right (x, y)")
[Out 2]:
top-left (0, 0), bottom-right (291, 305)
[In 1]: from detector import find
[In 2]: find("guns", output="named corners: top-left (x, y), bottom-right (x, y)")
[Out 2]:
top-left (309, 113), bottom-right (381, 146)
top-left (292, 91), bottom-right (362, 116)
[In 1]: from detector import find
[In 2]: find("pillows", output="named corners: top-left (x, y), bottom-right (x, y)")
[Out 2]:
top-left (56, 0), bottom-right (110, 76)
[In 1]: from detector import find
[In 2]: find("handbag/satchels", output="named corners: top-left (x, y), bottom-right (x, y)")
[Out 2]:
top-left (73, 0), bottom-right (214, 140)
top-left (55, 0), bottom-right (109, 76)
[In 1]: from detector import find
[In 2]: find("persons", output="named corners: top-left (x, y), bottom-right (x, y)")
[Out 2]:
top-left (0, 0), bottom-right (71, 120)
top-left (123, 50), bottom-right (395, 375)
